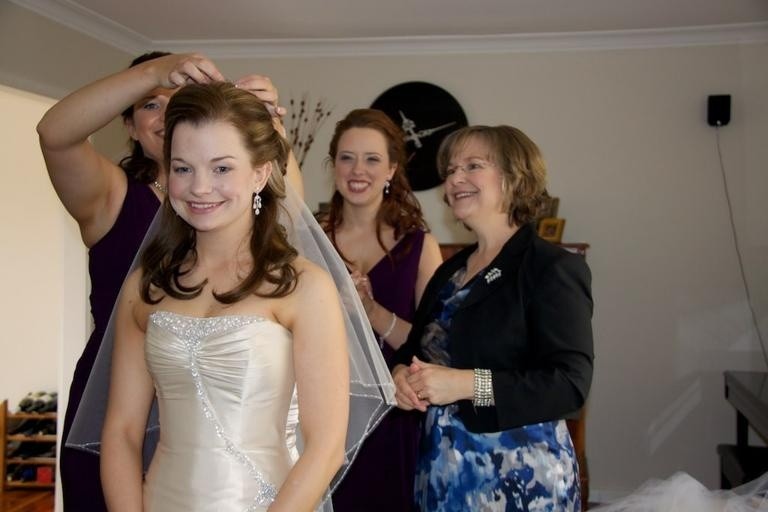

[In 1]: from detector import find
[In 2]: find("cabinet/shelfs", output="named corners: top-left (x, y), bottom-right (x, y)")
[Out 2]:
top-left (0, 398), bottom-right (57, 491)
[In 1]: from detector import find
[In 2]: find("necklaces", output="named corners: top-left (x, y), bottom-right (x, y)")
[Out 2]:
top-left (152, 180), bottom-right (167, 194)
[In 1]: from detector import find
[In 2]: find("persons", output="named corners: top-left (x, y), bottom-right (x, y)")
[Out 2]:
top-left (392, 124), bottom-right (595, 511)
top-left (35, 50), bottom-right (306, 512)
top-left (64, 81), bottom-right (399, 512)
top-left (311, 106), bottom-right (445, 511)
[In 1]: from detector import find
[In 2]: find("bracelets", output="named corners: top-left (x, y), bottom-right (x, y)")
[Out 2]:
top-left (473, 368), bottom-right (495, 408)
top-left (379, 312), bottom-right (397, 349)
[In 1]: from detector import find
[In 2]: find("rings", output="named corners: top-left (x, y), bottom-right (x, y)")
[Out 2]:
top-left (417, 391), bottom-right (421, 400)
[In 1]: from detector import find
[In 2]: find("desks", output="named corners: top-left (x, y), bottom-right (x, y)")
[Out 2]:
top-left (719, 369), bottom-right (767, 486)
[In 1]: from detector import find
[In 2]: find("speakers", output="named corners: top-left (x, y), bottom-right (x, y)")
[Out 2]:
top-left (708, 95), bottom-right (730, 126)
